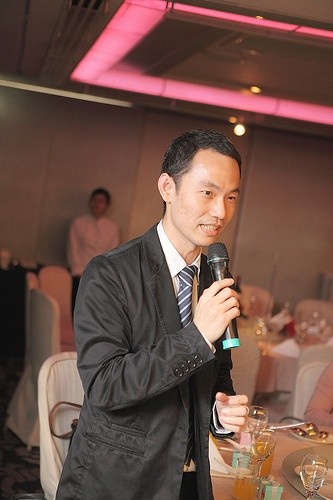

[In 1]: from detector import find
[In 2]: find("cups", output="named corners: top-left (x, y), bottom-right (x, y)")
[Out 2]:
top-left (232, 456), bottom-right (256, 500)
top-left (255, 312), bottom-right (331, 346)
top-left (252, 442), bottom-right (275, 479)
top-left (246, 405), bottom-right (269, 440)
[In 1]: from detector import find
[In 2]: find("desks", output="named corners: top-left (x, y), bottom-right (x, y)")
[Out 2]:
top-left (232, 323), bottom-right (333, 423)
top-left (270, 421), bottom-right (333, 500)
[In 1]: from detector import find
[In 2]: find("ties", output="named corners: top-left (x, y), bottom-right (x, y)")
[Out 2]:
top-left (177, 264), bottom-right (194, 467)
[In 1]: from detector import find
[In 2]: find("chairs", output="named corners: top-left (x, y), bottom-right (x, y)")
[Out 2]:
top-left (3, 287), bottom-right (62, 452)
top-left (207, 434), bottom-right (264, 500)
top-left (298, 341), bottom-right (333, 367)
top-left (239, 284), bottom-right (276, 319)
top-left (229, 316), bottom-right (262, 407)
top-left (23, 271), bottom-right (37, 365)
top-left (39, 264), bottom-right (76, 353)
top-left (38, 353), bottom-right (85, 500)
top-left (293, 297), bottom-right (333, 328)
top-left (293, 361), bottom-right (328, 423)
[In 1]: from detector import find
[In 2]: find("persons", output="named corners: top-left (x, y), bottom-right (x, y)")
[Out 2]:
top-left (66, 189), bottom-right (121, 330)
top-left (56, 129), bottom-right (249, 500)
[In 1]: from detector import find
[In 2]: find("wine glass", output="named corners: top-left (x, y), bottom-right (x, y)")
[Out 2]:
top-left (251, 429), bottom-right (277, 480)
top-left (300, 454), bottom-right (328, 500)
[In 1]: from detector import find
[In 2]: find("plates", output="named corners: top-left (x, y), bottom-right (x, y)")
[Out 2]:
top-left (289, 426), bottom-right (333, 444)
top-left (294, 465), bottom-right (333, 500)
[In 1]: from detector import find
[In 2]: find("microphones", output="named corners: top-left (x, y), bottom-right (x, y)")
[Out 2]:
top-left (209, 241), bottom-right (240, 349)
